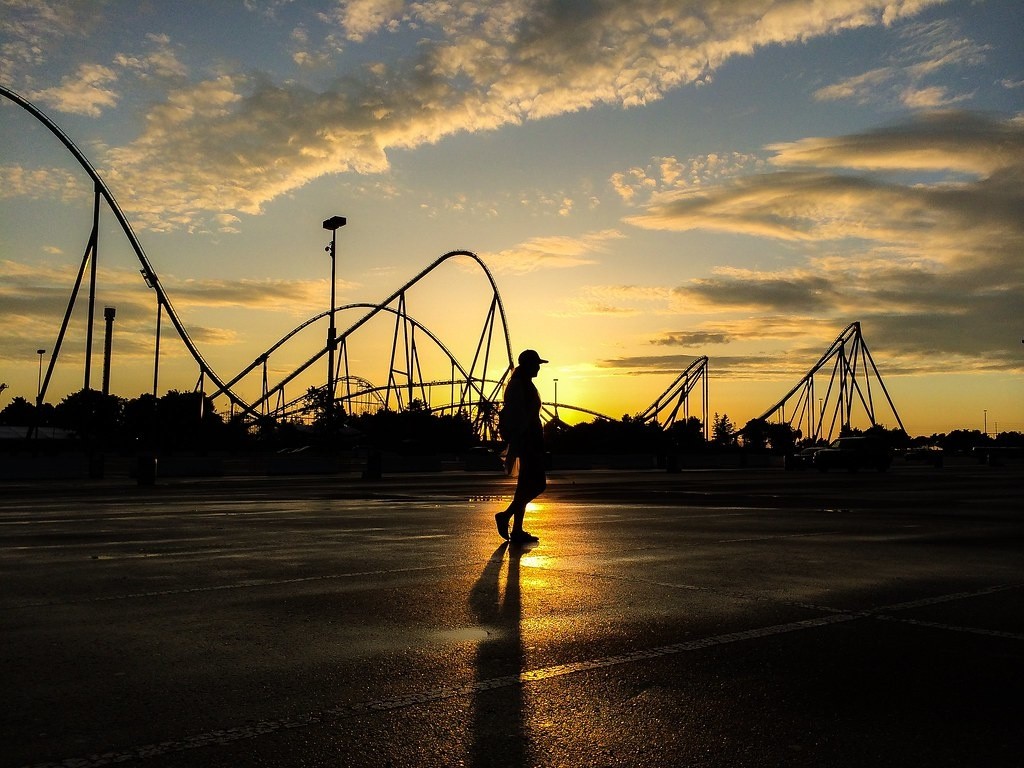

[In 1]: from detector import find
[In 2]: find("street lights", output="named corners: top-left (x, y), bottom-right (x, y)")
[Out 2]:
top-left (553, 379), bottom-right (558, 417)
top-left (37, 349), bottom-right (45, 397)
top-left (819, 398), bottom-right (823, 438)
top-left (984, 410), bottom-right (987, 433)
top-left (320, 216), bottom-right (347, 419)
top-left (102, 305), bottom-right (118, 404)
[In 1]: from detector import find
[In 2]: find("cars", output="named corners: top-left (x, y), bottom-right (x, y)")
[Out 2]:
top-left (904, 448), bottom-right (928, 462)
top-left (794, 447), bottom-right (828, 469)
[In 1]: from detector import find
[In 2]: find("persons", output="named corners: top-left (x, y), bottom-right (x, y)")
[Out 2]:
top-left (495, 350), bottom-right (549, 542)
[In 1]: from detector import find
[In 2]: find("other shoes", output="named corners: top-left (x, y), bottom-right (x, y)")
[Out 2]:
top-left (511, 530), bottom-right (539, 542)
top-left (495, 512), bottom-right (509, 540)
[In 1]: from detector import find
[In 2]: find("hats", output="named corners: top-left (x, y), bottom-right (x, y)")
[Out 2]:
top-left (519, 349), bottom-right (548, 364)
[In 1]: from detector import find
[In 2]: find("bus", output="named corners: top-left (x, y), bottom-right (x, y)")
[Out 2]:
top-left (972, 446), bottom-right (1024, 465)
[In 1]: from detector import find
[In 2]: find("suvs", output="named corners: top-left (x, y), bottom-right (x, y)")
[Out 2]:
top-left (812, 437), bottom-right (894, 474)
